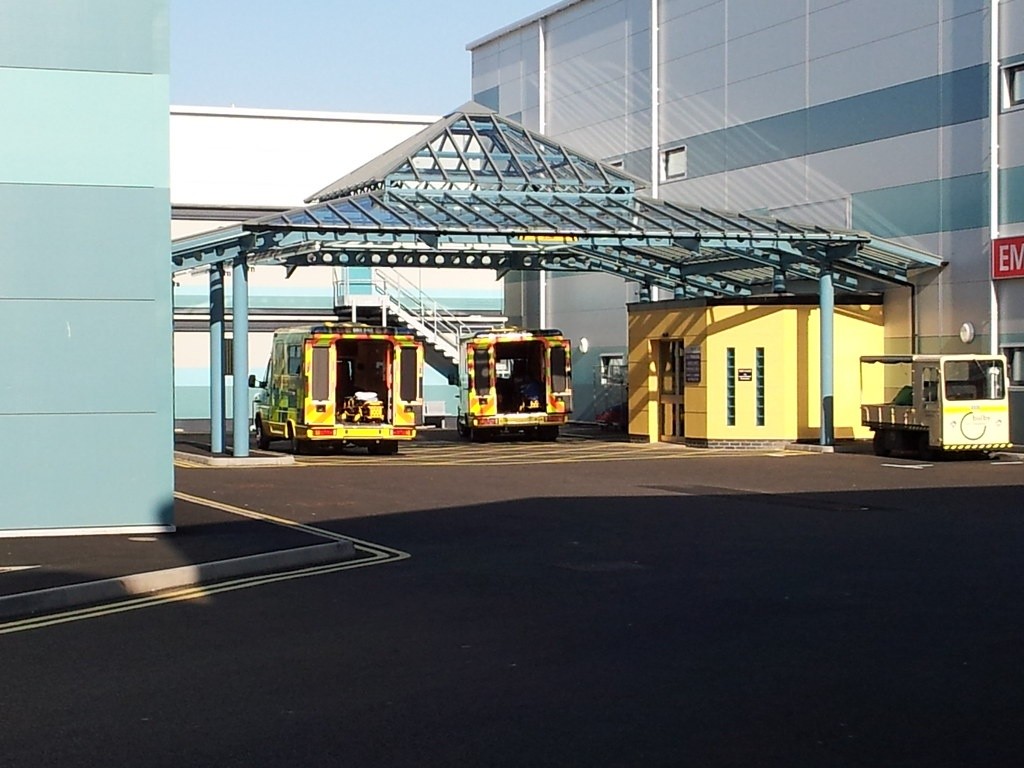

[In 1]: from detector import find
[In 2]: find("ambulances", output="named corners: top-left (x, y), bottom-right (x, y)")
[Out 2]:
top-left (249, 320), bottom-right (426, 453)
top-left (457, 327), bottom-right (574, 443)
